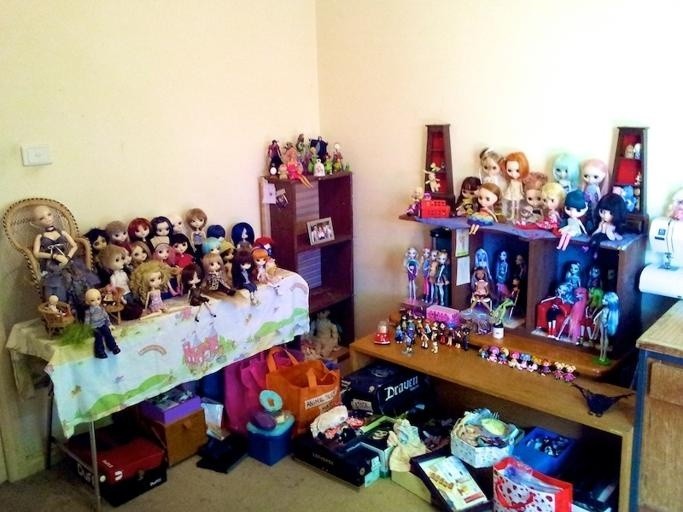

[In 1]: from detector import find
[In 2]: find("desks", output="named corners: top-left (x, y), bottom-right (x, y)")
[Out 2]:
top-left (5, 266), bottom-right (312, 510)
top-left (347, 327), bottom-right (637, 512)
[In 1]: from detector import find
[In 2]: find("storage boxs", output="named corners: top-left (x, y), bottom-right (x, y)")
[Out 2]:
top-left (409, 447), bottom-right (492, 512)
top-left (111, 388), bottom-right (206, 468)
top-left (55, 423), bottom-right (169, 508)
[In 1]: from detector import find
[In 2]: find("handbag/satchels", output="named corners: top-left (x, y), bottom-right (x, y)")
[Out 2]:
top-left (490, 456), bottom-right (574, 512)
top-left (223, 341), bottom-right (342, 438)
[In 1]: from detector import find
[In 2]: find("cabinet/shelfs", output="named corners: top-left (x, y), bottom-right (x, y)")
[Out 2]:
top-left (423, 122), bottom-right (455, 212)
top-left (634, 300), bottom-right (683, 512)
top-left (608, 125), bottom-right (651, 233)
top-left (259, 171), bottom-right (355, 364)
top-left (398, 209), bottom-right (649, 387)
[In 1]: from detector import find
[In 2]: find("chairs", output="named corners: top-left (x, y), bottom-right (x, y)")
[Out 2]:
top-left (1, 198), bottom-right (124, 328)
top-left (34, 297), bottom-right (76, 338)
top-left (94, 282), bottom-right (124, 324)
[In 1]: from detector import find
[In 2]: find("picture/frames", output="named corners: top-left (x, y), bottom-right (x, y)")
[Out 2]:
top-left (304, 216), bottom-right (335, 247)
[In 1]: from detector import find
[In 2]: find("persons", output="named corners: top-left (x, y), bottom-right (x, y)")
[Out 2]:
top-left (261, 130), bottom-right (350, 189)
top-left (305, 306), bottom-right (341, 356)
top-left (310, 224), bottom-right (332, 242)
